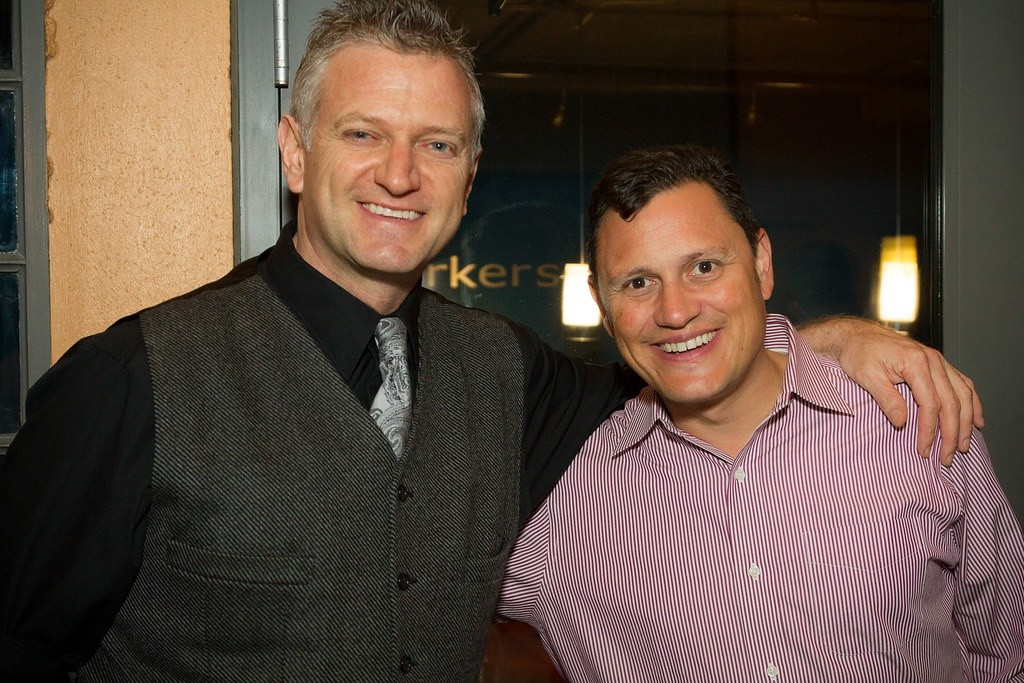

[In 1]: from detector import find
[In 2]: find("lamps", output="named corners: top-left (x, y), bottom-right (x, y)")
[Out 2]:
top-left (561, 7), bottom-right (604, 327)
top-left (875, 22), bottom-right (922, 327)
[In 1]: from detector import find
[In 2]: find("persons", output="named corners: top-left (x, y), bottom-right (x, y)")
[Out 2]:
top-left (0, 0), bottom-right (988, 682)
top-left (490, 140), bottom-right (1023, 683)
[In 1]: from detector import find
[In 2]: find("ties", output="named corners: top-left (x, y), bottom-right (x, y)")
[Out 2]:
top-left (369, 316), bottom-right (412, 459)
